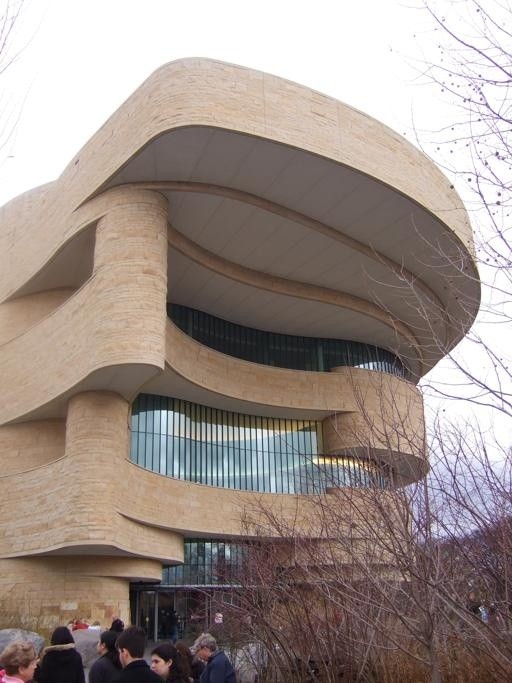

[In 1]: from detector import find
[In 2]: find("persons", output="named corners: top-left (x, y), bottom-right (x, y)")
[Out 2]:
top-left (436, 590), bottom-right (511, 631)
top-left (0, 606), bottom-right (253, 683)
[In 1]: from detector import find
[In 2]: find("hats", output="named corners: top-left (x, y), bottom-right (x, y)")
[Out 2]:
top-left (51, 627), bottom-right (74, 644)
